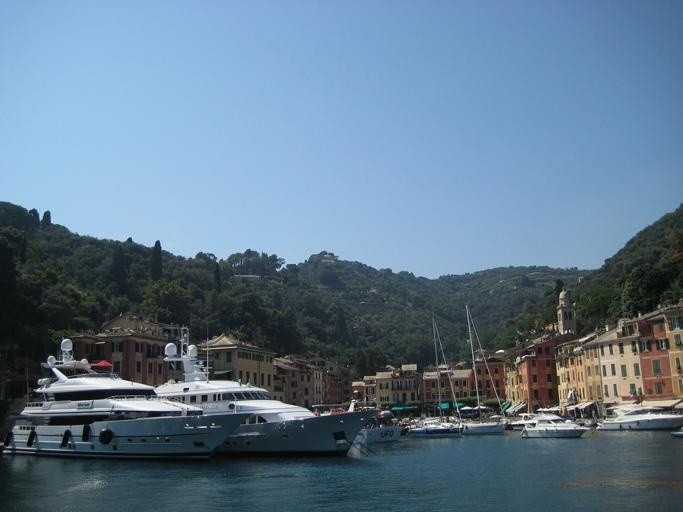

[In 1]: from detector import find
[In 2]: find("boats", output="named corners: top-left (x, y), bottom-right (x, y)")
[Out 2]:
top-left (597, 408), bottom-right (683, 431)
top-left (348, 401), bottom-right (402, 445)
top-left (509, 414), bottom-right (590, 438)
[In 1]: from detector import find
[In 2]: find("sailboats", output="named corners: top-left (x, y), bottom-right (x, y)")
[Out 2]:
top-left (409, 304), bottom-right (509, 435)
